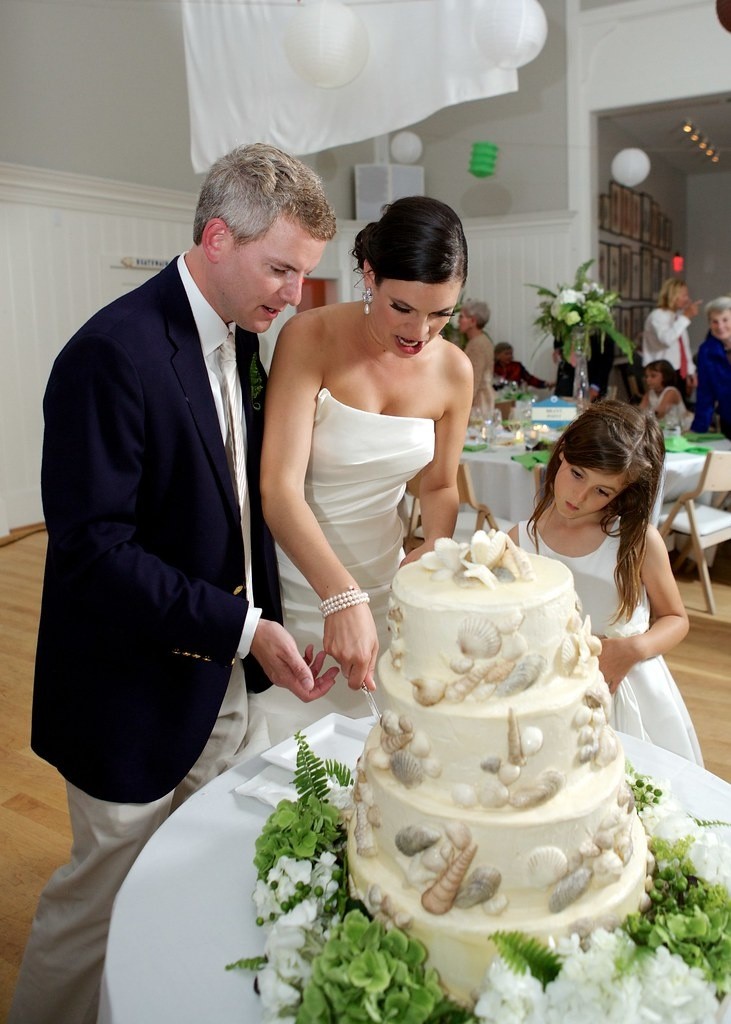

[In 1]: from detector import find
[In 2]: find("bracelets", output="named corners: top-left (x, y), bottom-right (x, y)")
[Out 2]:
top-left (318, 585), bottom-right (370, 619)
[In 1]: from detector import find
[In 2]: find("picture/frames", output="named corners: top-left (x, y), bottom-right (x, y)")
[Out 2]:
top-left (598, 181), bottom-right (675, 356)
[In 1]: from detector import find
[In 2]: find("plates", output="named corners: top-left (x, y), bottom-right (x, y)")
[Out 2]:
top-left (260, 713), bottom-right (374, 780)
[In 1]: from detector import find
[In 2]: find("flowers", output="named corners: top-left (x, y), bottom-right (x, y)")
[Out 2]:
top-left (478, 928), bottom-right (722, 1024)
top-left (325, 775), bottom-right (352, 810)
top-left (529, 257), bottom-right (635, 363)
top-left (254, 853), bottom-right (339, 1024)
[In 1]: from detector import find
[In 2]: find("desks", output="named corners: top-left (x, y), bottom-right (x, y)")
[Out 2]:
top-left (102, 737), bottom-right (731, 1024)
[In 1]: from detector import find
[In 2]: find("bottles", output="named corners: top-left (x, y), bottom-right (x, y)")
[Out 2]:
top-left (508, 381), bottom-right (517, 390)
top-left (589, 384), bottom-right (599, 403)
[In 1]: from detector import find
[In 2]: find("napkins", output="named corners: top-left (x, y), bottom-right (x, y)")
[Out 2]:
top-left (235, 765), bottom-right (302, 809)
top-left (511, 450), bottom-right (552, 470)
top-left (664, 435), bottom-right (712, 454)
top-left (685, 432), bottom-right (724, 441)
top-left (464, 444), bottom-right (489, 452)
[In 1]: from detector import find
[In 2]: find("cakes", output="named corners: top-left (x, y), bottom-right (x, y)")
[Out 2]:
top-left (343, 529), bottom-right (655, 1019)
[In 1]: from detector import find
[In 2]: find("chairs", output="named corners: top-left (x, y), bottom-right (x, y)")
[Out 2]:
top-left (404, 462), bottom-right (515, 557)
top-left (657, 451), bottom-right (731, 615)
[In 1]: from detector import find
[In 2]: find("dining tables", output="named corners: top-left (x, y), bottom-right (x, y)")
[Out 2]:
top-left (453, 429), bottom-right (731, 568)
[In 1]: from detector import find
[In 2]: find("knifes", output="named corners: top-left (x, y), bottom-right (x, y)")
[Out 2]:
top-left (360, 682), bottom-right (382, 722)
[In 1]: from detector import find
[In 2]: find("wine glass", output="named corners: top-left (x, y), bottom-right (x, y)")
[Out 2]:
top-left (491, 376), bottom-right (505, 402)
top-left (483, 416), bottom-right (493, 439)
top-left (508, 416), bottom-right (520, 445)
top-left (664, 416), bottom-right (682, 447)
top-left (470, 406), bottom-right (483, 445)
top-left (523, 427), bottom-right (540, 452)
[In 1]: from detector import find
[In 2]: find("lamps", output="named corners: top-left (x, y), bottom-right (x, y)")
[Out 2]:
top-left (684, 118), bottom-right (722, 163)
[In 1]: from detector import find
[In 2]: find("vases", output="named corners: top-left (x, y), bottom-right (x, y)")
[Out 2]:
top-left (572, 328), bottom-right (589, 405)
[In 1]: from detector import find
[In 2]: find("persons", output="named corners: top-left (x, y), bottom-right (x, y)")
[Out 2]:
top-left (5, 143), bottom-right (341, 1024)
top-left (633, 275), bottom-right (731, 442)
top-left (259, 195), bottom-right (474, 749)
top-left (457, 300), bottom-right (495, 428)
top-left (493, 326), bottom-right (616, 403)
top-left (507, 401), bottom-right (705, 769)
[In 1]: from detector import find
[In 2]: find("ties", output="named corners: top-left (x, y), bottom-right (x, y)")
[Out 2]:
top-left (219, 332), bottom-right (248, 519)
top-left (675, 314), bottom-right (688, 379)
